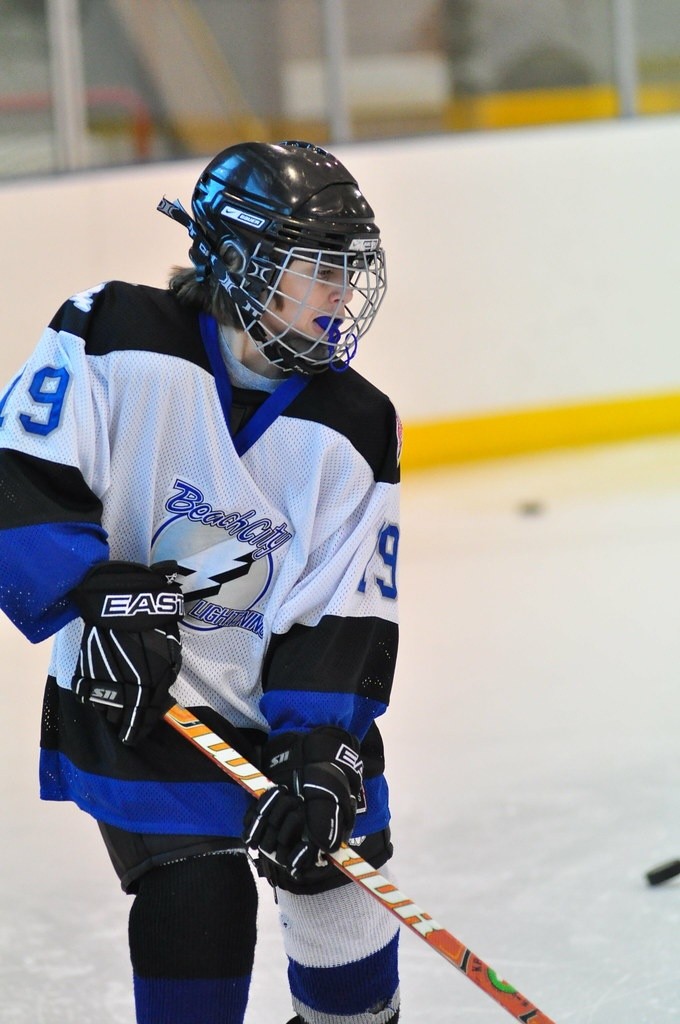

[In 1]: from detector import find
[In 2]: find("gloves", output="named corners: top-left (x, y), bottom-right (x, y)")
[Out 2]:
top-left (241, 726), bottom-right (364, 882)
top-left (70, 560), bottom-right (183, 746)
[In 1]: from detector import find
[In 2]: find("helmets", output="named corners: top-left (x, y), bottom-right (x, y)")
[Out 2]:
top-left (157, 139), bottom-right (383, 376)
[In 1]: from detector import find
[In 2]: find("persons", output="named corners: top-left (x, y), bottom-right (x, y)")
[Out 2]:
top-left (1, 139), bottom-right (403, 1023)
